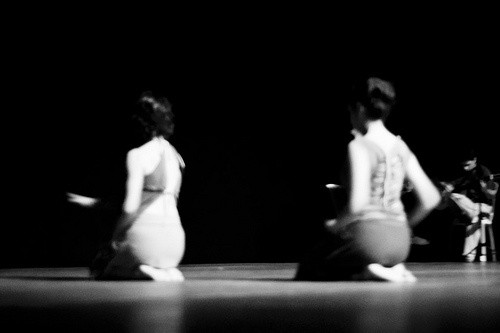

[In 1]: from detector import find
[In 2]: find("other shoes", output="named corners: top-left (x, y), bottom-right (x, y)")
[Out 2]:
top-left (138, 265), bottom-right (184, 282)
top-left (364, 262), bottom-right (411, 282)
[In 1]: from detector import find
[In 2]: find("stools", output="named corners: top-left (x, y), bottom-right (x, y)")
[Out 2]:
top-left (479, 216), bottom-right (497, 263)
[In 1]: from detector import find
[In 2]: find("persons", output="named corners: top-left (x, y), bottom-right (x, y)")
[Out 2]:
top-left (440, 150), bottom-right (498, 263)
top-left (297, 78), bottom-right (442, 285)
top-left (89, 96), bottom-right (186, 282)
